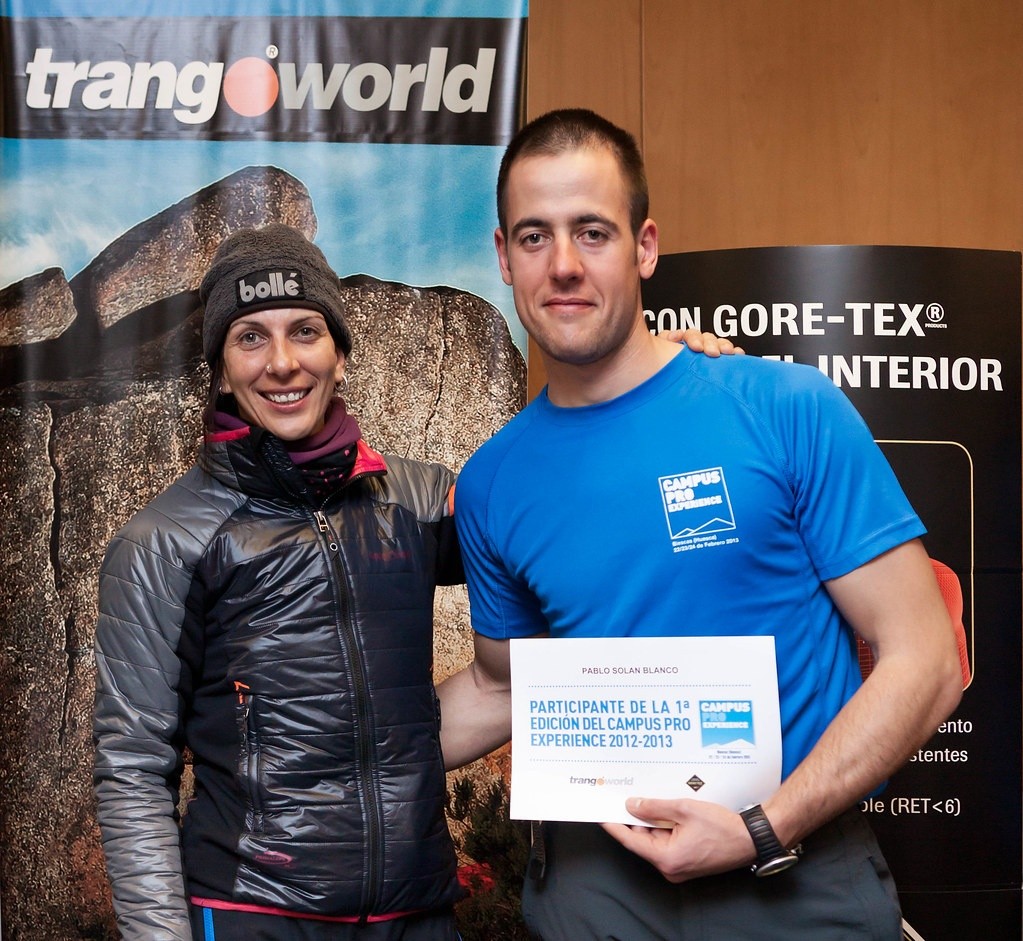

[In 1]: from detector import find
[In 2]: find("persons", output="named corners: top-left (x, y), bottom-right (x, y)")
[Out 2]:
top-left (432, 107), bottom-right (967, 941)
top-left (85, 218), bottom-right (744, 940)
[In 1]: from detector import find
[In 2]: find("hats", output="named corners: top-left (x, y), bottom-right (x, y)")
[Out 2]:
top-left (199, 222), bottom-right (352, 371)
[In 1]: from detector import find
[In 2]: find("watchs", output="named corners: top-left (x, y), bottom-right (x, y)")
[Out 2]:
top-left (739, 802), bottom-right (804, 880)
